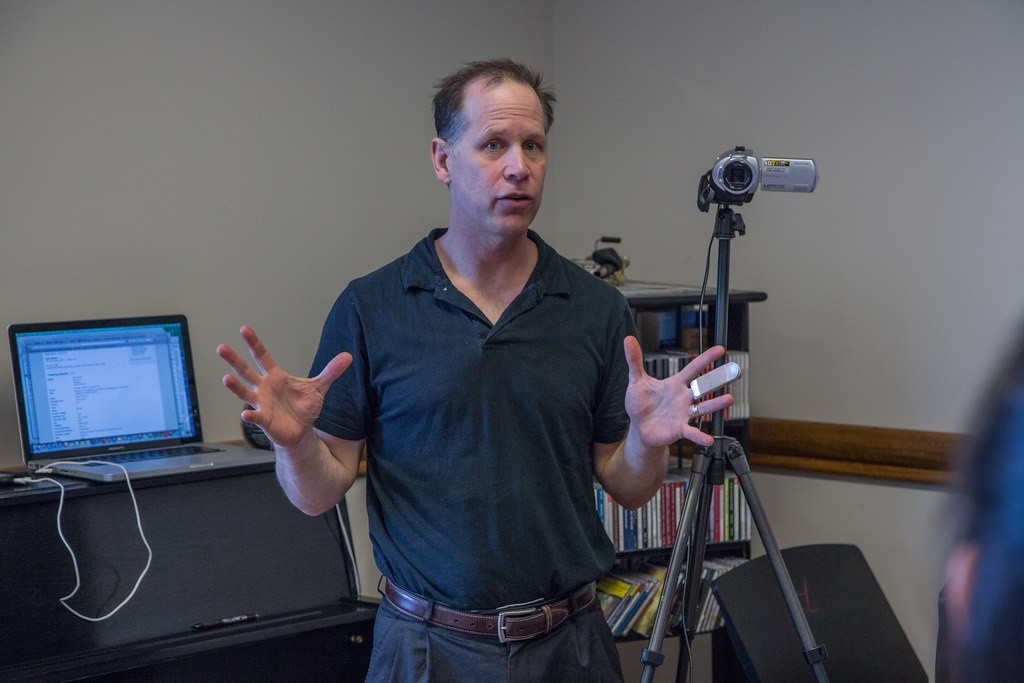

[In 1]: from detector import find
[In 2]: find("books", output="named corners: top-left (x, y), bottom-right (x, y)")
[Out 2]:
top-left (595, 559), bottom-right (749, 638)
top-left (641, 350), bottom-right (749, 422)
top-left (592, 466), bottom-right (752, 553)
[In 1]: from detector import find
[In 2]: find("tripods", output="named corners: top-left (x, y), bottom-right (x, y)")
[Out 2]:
top-left (640, 202), bottom-right (832, 683)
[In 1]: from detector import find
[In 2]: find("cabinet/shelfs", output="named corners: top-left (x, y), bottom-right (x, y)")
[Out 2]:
top-left (592, 280), bottom-right (769, 645)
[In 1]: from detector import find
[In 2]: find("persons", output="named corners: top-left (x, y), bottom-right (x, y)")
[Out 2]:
top-left (217, 58), bottom-right (742, 683)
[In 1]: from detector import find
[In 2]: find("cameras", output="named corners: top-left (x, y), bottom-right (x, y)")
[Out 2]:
top-left (710, 144), bottom-right (817, 206)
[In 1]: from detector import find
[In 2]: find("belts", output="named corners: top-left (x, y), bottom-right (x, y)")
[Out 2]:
top-left (385, 577), bottom-right (598, 644)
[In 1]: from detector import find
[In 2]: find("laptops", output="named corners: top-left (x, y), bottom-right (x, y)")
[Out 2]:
top-left (8, 314), bottom-right (277, 483)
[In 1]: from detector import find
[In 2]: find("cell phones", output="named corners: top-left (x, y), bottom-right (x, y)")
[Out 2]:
top-left (0, 472), bottom-right (14, 484)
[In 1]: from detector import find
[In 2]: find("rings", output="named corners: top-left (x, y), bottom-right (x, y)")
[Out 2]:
top-left (692, 403), bottom-right (703, 417)
